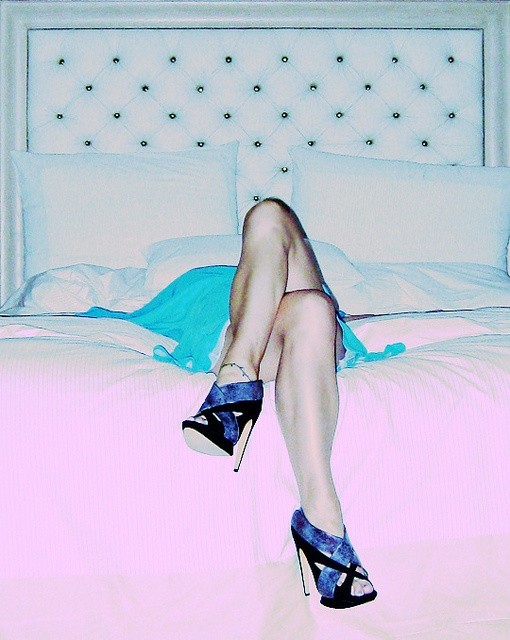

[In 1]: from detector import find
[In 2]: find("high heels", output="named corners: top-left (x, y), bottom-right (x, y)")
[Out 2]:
top-left (180, 380), bottom-right (263, 473)
top-left (291, 508), bottom-right (378, 609)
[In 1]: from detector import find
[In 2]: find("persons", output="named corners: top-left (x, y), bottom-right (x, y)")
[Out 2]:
top-left (151, 197), bottom-right (377, 610)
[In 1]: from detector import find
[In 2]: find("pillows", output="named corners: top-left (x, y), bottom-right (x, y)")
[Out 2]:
top-left (140, 235), bottom-right (363, 288)
top-left (9, 139), bottom-right (241, 280)
top-left (287, 145), bottom-right (509, 272)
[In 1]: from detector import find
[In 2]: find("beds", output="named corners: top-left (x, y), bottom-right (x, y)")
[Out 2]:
top-left (1, 0), bottom-right (510, 633)
